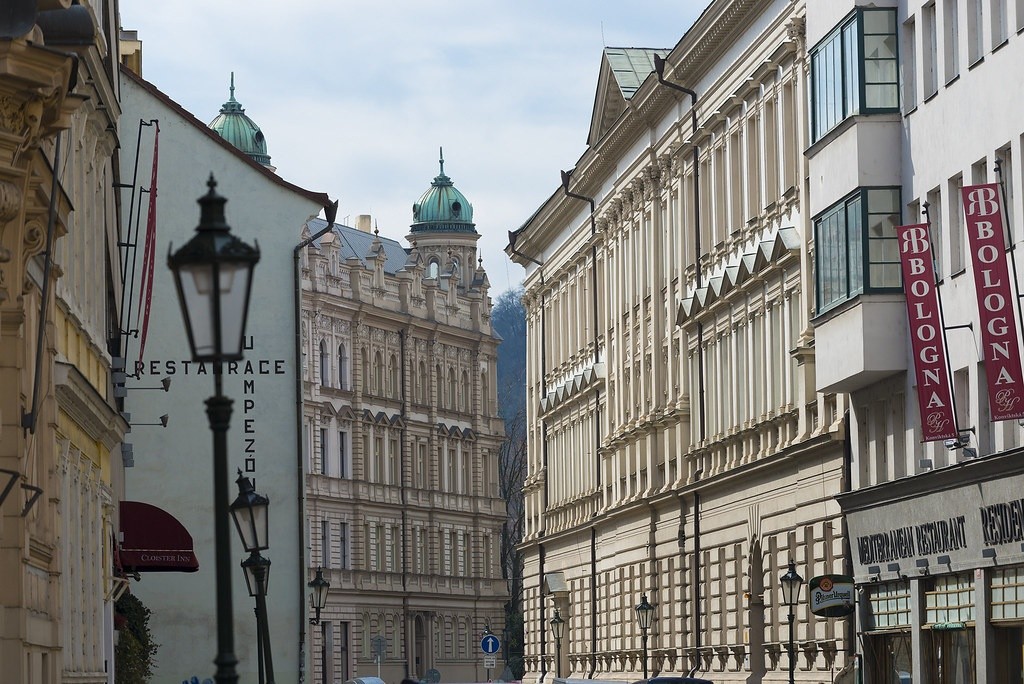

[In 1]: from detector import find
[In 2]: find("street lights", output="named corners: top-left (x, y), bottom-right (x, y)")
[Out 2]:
top-left (224, 469), bottom-right (269, 684)
top-left (164, 172), bottom-right (261, 684)
top-left (550, 610), bottom-right (565, 677)
top-left (482, 625), bottom-right (494, 682)
top-left (781, 559), bottom-right (803, 684)
top-left (634, 593), bottom-right (654, 679)
top-left (242, 552), bottom-right (274, 684)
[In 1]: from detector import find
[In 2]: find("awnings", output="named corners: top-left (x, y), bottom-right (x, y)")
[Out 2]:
top-left (118, 500), bottom-right (200, 572)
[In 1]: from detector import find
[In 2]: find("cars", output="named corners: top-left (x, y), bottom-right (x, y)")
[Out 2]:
top-left (344, 677), bottom-right (386, 684)
